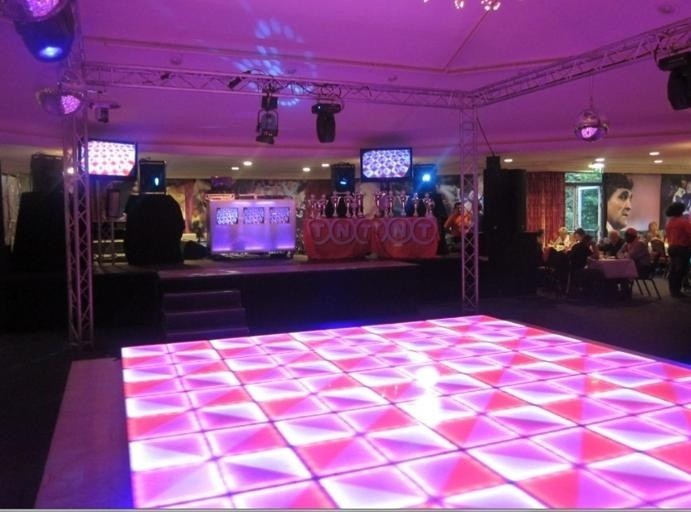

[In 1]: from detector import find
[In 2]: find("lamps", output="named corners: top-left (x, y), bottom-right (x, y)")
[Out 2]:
top-left (255, 94), bottom-right (279, 145)
top-left (14, 6), bottom-right (75, 63)
top-left (312, 99), bottom-right (342, 143)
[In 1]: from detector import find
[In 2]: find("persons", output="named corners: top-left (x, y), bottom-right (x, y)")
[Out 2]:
top-left (660, 176), bottom-right (691, 227)
top-left (535, 201), bottom-right (691, 299)
top-left (603, 173), bottom-right (634, 231)
top-left (443, 201), bottom-right (473, 252)
top-left (470, 202), bottom-right (484, 222)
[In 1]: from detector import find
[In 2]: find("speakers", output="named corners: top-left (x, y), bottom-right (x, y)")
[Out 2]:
top-left (138, 160), bottom-right (167, 195)
top-left (331, 163), bottom-right (354, 191)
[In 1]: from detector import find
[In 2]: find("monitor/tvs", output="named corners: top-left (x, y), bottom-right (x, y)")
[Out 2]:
top-left (77, 137), bottom-right (137, 181)
top-left (359, 147), bottom-right (412, 181)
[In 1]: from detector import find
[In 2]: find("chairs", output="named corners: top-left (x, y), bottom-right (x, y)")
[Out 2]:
top-left (538, 237), bottom-right (667, 299)
